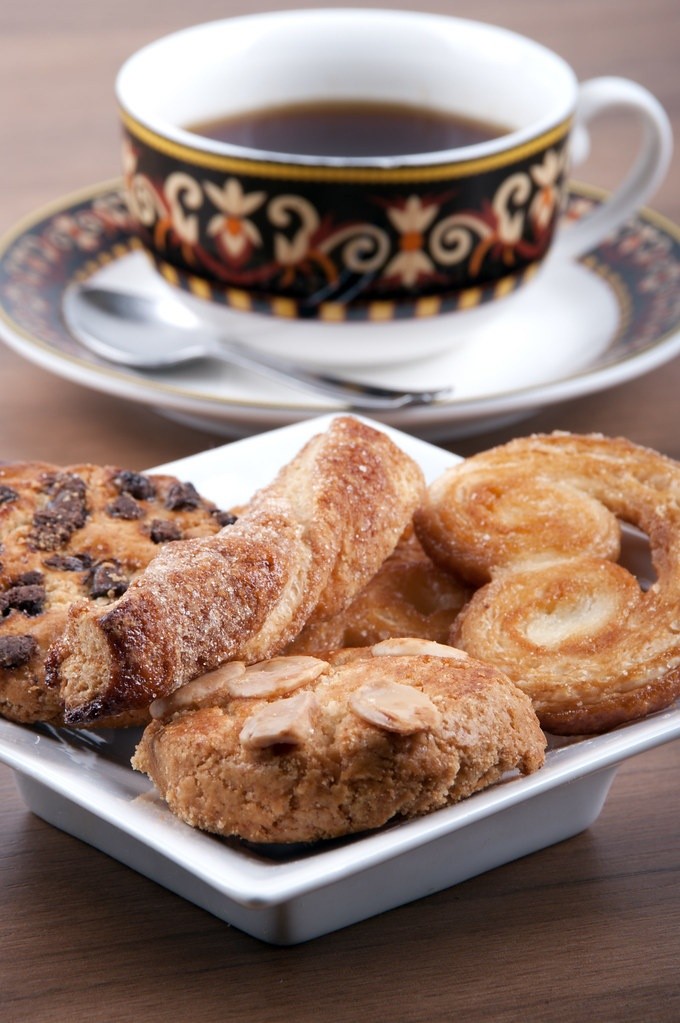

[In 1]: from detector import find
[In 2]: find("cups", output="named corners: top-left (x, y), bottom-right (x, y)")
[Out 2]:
top-left (111, 9), bottom-right (672, 366)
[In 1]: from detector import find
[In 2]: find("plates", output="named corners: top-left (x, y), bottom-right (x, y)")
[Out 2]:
top-left (4, 412), bottom-right (680, 943)
top-left (3, 182), bottom-right (680, 438)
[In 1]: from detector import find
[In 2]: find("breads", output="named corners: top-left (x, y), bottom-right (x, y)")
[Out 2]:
top-left (0, 416), bottom-right (680, 842)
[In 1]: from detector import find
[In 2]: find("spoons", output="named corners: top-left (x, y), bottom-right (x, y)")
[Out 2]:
top-left (60, 281), bottom-right (450, 414)
